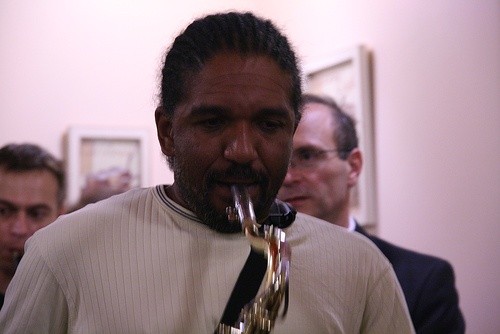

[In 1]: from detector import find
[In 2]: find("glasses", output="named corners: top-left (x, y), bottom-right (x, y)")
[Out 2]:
top-left (287, 146), bottom-right (355, 172)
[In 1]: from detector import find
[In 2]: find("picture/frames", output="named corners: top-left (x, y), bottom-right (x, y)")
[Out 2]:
top-left (299, 44), bottom-right (375, 229)
top-left (62, 125), bottom-right (149, 205)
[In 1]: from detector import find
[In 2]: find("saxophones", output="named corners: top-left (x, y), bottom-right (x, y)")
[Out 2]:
top-left (212, 182), bottom-right (294, 334)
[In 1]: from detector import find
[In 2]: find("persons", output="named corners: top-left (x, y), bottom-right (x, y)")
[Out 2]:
top-left (0, 13), bottom-right (420, 334)
top-left (276, 95), bottom-right (467, 334)
top-left (0, 143), bottom-right (64, 309)
